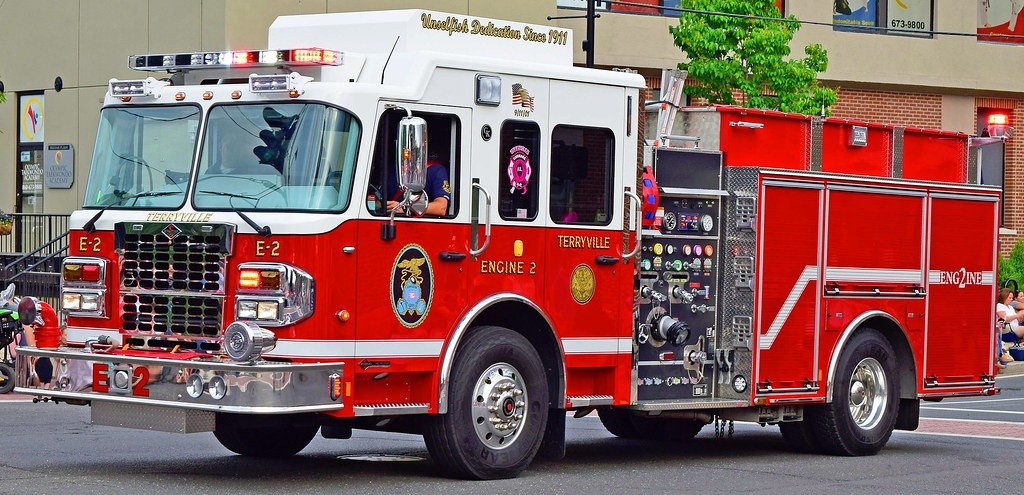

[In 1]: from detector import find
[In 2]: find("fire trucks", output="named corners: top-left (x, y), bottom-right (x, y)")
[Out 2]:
top-left (13, 7), bottom-right (1004, 480)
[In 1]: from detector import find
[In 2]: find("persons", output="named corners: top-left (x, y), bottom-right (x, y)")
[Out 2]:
top-left (204, 132), bottom-right (249, 175)
top-left (369, 148), bottom-right (451, 216)
top-left (996, 288), bottom-right (1024, 369)
top-left (0, 283), bottom-right (66, 389)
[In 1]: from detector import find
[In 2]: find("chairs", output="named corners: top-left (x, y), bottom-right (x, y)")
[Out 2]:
top-left (997, 317), bottom-right (1024, 348)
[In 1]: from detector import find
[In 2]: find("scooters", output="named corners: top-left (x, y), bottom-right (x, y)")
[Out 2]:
top-left (0, 309), bottom-right (24, 393)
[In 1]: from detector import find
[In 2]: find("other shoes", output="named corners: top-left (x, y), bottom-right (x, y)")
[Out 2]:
top-left (1000, 355), bottom-right (1014, 363)
top-left (996, 361), bottom-right (1005, 369)
